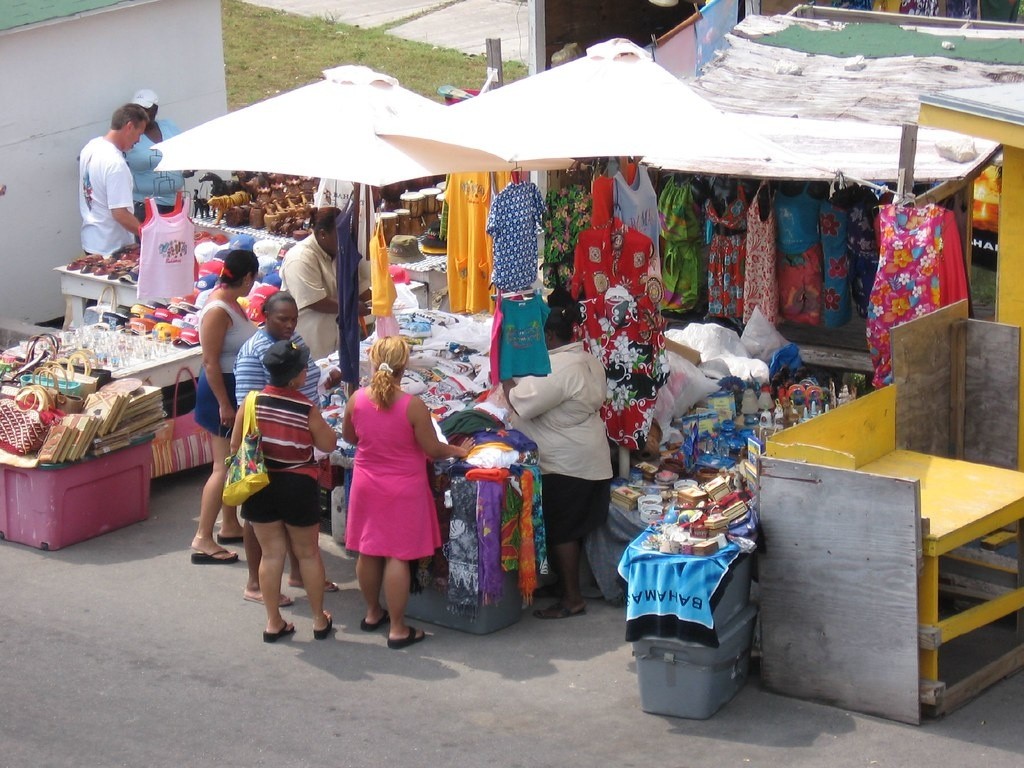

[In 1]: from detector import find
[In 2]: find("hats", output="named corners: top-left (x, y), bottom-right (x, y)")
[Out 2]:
top-left (263, 340), bottom-right (310, 387)
top-left (422, 245), bottom-right (446, 254)
top-left (66, 231), bottom-right (296, 345)
top-left (130, 89), bottom-right (159, 108)
top-left (387, 235), bottom-right (426, 264)
top-left (420, 220), bottom-right (447, 247)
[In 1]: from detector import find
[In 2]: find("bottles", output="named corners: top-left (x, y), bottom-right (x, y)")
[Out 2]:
top-left (743, 381), bottom-right (774, 429)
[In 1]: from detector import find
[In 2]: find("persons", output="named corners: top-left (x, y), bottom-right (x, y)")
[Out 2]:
top-left (79, 88), bottom-right (196, 309)
top-left (501, 305), bottom-right (614, 617)
top-left (341, 337), bottom-right (476, 650)
top-left (188, 207), bottom-right (344, 641)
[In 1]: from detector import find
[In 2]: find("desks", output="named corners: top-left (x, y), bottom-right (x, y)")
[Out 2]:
top-left (855, 447), bottom-right (1024, 720)
top-left (4, 206), bottom-right (511, 485)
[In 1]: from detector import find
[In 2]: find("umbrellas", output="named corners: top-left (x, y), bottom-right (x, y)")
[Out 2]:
top-left (146, 63), bottom-right (580, 244)
top-left (373, 37), bottom-right (841, 179)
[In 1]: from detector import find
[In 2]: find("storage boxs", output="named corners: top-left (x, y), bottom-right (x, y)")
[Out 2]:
top-left (632, 601), bottom-right (756, 720)
top-left (0, 432), bottom-right (156, 551)
top-left (713, 550), bottom-right (755, 631)
top-left (379, 563), bottom-right (522, 634)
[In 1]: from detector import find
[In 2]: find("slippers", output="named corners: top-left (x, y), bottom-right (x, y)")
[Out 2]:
top-left (534, 603), bottom-right (587, 618)
top-left (533, 584), bottom-right (563, 598)
top-left (387, 626), bottom-right (425, 648)
top-left (362, 608), bottom-right (390, 630)
top-left (314, 615), bottom-right (332, 639)
top-left (217, 534), bottom-right (243, 544)
top-left (191, 550), bottom-right (239, 564)
top-left (263, 620), bottom-right (294, 643)
top-left (243, 593), bottom-right (293, 606)
top-left (289, 580), bottom-right (337, 592)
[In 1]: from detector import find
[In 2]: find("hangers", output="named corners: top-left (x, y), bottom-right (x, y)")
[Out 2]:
top-left (580, 157), bottom-right (656, 312)
top-left (335, 183), bottom-right (358, 228)
top-left (491, 286), bottom-right (545, 302)
top-left (376, 186), bottom-right (382, 231)
top-left (133, 149), bottom-right (192, 209)
top-left (872, 192), bottom-right (949, 214)
top-left (497, 161), bottom-right (535, 197)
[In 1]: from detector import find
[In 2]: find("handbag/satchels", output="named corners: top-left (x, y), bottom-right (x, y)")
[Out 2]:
top-left (221, 391), bottom-right (269, 506)
top-left (150, 366), bottom-right (216, 479)
top-left (83, 285), bottom-right (129, 327)
top-left (0, 333), bottom-right (111, 456)
